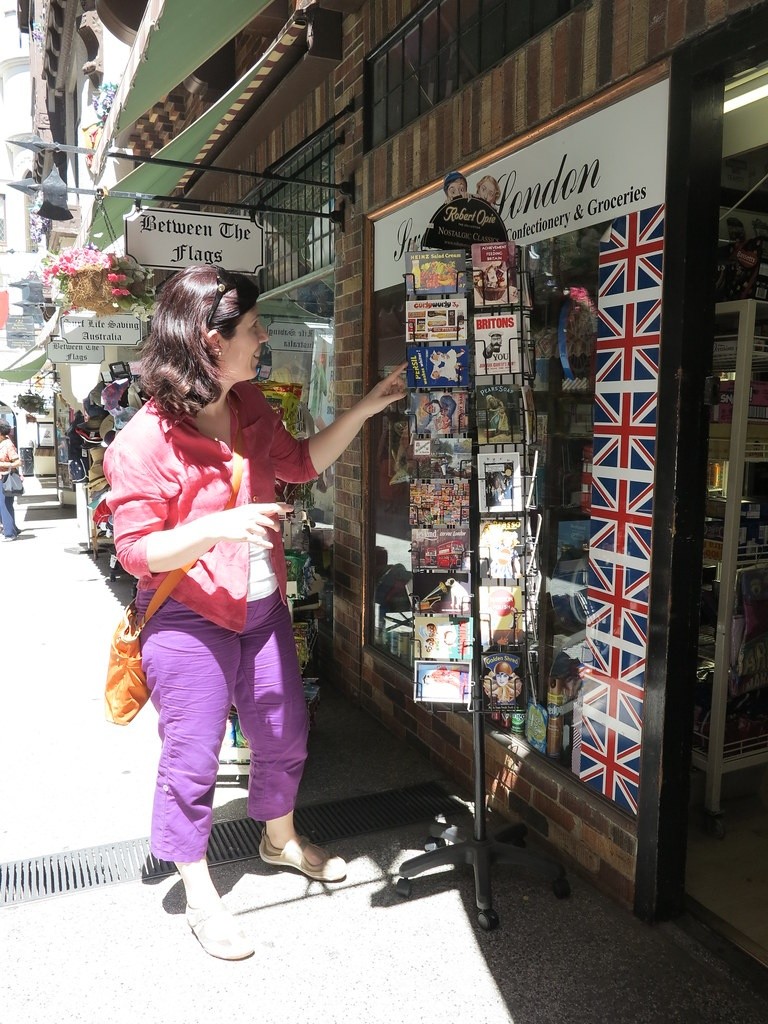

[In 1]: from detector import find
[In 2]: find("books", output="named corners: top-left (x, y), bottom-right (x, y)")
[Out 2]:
top-left (407, 242), bottom-right (528, 708)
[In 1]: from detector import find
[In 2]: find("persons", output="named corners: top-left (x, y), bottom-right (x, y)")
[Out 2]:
top-left (498, 467), bottom-right (513, 506)
top-left (474, 176), bottom-right (501, 211)
top-left (103, 265), bottom-right (409, 961)
top-left (484, 662), bottom-right (522, 707)
top-left (443, 172), bottom-right (470, 204)
top-left (483, 330), bottom-right (503, 359)
top-left (423, 667), bottom-right (469, 687)
top-left (0, 419), bottom-right (22, 542)
top-left (487, 531), bottom-right (522, 579)
top-left (486, 395), bottom-right (509, 436)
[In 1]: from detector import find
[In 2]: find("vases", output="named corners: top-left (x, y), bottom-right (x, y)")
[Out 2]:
top-left (67, 271), bottom-right (123, 316)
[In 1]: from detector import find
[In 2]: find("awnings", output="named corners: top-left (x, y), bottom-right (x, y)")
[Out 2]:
top-left (72, 0), bottom-right (307, 253)
top-left (0, 346), bottom-right (47, 384)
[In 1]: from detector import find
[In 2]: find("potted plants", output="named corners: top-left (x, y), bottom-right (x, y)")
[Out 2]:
top-left (14, 389), bottom-right (50, 414)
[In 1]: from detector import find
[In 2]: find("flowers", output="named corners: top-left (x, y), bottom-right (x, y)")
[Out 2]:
top-left (39, 239), bottom-right (161, 322)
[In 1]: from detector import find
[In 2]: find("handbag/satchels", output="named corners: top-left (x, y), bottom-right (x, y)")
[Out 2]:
top-left (2, 468), bottom-right (24, 498)
top-left (106, 597), bottom-right (152, 725)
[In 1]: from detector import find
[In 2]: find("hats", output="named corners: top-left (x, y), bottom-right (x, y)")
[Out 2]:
top-left (65, 378), bottom-right (144, 493)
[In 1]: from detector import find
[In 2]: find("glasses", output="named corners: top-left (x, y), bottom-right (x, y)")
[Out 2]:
top-left (204, 263), bottom-right (237, 329)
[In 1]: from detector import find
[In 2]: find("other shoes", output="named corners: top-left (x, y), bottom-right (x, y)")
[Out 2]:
top-left (1, 535), bottom-right (16, 542)
top-left (259, 825), bottom-right (347, 881)
top-left (185, 898), bottom-right (254, 960)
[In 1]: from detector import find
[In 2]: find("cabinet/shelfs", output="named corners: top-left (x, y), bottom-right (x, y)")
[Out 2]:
top-left (394, 272), bottom-right (573, 931)
top-left (686, 298), bottom-right (768, 840)
top-left (20, 447), bottom-right (34, 475)
